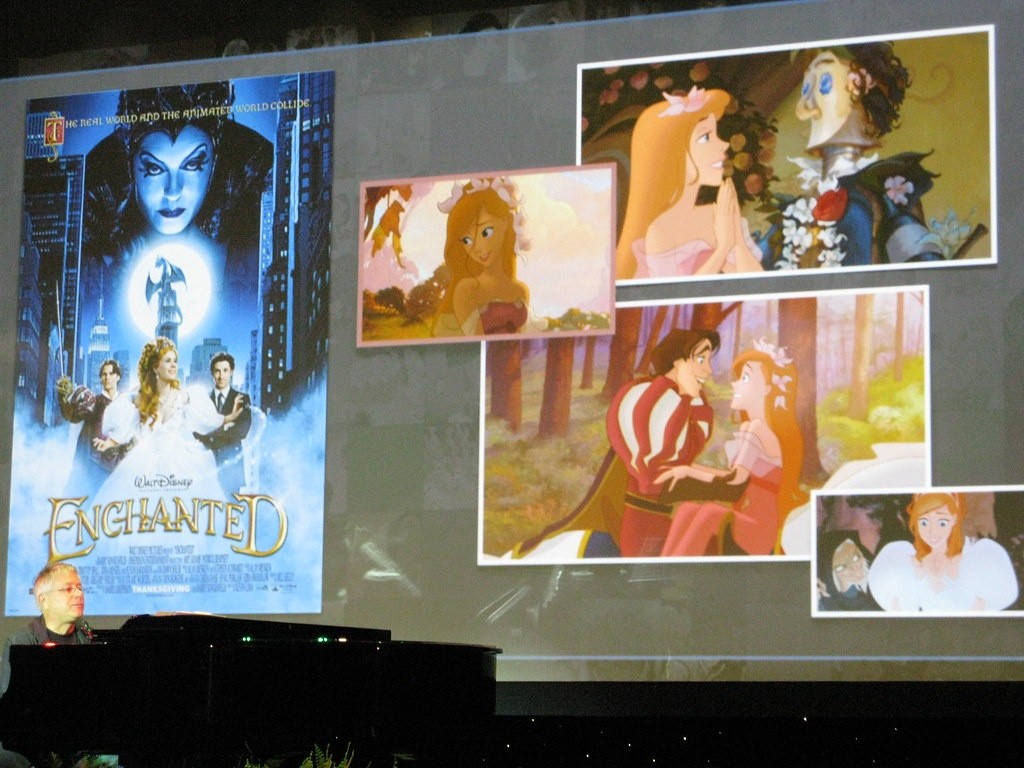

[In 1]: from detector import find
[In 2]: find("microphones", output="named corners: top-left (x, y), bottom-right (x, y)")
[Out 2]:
top-left (77, 619), bottom-right (93, 639)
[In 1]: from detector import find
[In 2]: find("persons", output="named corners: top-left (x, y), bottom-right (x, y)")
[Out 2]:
top-left (57, 339), bottom-right (252, 535)
top-left (0, 561), bottom-right (93, 696)
top-left (85, 78), bottom-right (275, 264)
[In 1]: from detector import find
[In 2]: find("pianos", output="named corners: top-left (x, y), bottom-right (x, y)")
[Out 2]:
top-left (0, 609), bottom-right (506, 768)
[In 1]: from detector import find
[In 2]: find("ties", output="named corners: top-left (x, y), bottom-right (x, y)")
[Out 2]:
top-left (218, 393), bottom-right (223, 414)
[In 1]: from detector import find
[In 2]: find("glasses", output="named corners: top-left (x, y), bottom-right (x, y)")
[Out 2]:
top-left (46, 585), bottom-right (83, 595)
